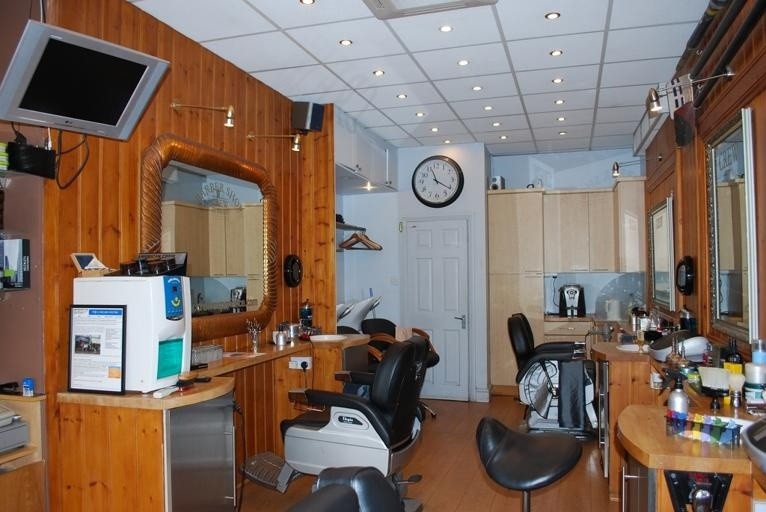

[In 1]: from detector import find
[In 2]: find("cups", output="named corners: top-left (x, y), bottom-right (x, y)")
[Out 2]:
top-left (287, 325), bottom-right (299, 339)
top-left (119, 255), bottom-right (176, 274)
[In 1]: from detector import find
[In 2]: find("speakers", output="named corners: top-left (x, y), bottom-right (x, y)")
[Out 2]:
top-left (292, 102), bottom-right (324, 131)
top-left (488, 176), bottom-right (505, 189)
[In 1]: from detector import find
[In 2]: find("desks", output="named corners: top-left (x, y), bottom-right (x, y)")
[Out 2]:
top-left (586, 333), bottom-right (765, 512)
top-left (55, 332), bottom-right (369, 512)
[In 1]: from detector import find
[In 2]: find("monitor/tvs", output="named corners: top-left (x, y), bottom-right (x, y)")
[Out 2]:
top-left (0, 19), bottom-right (170, 142)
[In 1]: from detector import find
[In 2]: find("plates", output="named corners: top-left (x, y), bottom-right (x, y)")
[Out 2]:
top-left (310, 334), bottom-right (346, 342)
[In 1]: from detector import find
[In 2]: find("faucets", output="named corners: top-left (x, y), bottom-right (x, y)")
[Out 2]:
top-left (299, 319), bottom-right (317, 332)
top-left (649, 305), bottom-right (661, 328)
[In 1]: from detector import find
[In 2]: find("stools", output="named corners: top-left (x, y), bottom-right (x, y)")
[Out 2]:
top-left (468, 415), bottom-right (583, 512)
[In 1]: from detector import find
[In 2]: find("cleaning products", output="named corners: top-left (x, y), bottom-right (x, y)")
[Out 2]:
top-left (658, 368), bottom-right (689, 413)
top-left (667, 336), bottom-right (690, 368)
top-left (276, 346), bottom-right (287, 351)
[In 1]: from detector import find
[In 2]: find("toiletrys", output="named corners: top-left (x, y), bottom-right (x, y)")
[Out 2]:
top-left (275, 323), bottom-right (288, 346)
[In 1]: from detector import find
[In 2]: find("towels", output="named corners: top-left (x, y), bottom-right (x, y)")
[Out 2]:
top-left (395, 326), bottom-right (413, 342)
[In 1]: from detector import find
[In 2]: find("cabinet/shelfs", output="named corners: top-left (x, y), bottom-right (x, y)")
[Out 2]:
top-left (161, 199), bottom-right (209, 277)
top-left (545, 315), bottom-right (592, 360)
top-left (205, 206), bottom-right (242, 276)
top-left (540, 187), bottom-right (617, 274)
top-left (236, 203), bottom-right (264, 311)
top-left (489, 189), bottom-right (545, 398)
top-left (332, 104), bottom-right (398, 196)
top-left (611, 175), bottom-right (645, 272)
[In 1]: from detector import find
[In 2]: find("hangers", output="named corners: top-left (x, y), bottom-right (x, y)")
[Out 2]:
top-left (336, 225), bottom-right (382, 250)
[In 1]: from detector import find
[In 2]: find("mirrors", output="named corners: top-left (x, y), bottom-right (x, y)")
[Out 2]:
top-left (136, 133), bottom-right (279, 345)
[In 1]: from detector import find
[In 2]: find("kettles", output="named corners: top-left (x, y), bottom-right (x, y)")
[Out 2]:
top-left (606, 299), bottom-right (623, 320)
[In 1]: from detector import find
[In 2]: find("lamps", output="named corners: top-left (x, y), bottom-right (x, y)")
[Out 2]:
top-left (170, 99), bottom-right (238, 129)
top-left (608, 153), bottom-right (663, 177)
top-left (247, 131), bottom-right (301, 151)
top-left (647, 69), bottom-right (734, 111)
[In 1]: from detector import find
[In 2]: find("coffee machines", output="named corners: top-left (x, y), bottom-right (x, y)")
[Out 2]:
top-left (559, 284), bottom-right (586, 318)
top-left (230, 286), bottom-right (246, 307)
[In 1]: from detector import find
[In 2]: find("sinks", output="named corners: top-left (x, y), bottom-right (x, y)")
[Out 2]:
top-left (616, 344), bottom-right (650, 352)
top-left (310, 335), bottom-right (347, 341)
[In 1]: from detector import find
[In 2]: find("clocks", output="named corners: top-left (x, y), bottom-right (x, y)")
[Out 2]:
top-left (411, 154), bottom-right (464, 208)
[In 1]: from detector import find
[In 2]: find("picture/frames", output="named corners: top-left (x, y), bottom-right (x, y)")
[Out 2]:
top-left (644, 189), bottom-right (675, 312)
top-left (702, 105), bottom-right (758, 344)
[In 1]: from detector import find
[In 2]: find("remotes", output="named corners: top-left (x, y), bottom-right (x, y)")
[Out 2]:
top-left (153, 386), bottom-right (179, 398)
top-left (194, 375), bottom-right (211, 382)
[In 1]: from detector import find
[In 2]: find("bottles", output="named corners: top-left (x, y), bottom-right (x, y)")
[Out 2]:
top-left (667, 377), bottom-right (689, 414)
top-left (704, 344), bottom-right (714, 365)
top-left (725, 336), bottom-right (744, 376)
top-left (22, 378), bottom-right (33, 397)
top-left (667, 335), bottom-right (689, 372)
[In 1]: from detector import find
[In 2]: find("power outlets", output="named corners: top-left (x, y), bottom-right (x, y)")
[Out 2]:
top-left (289, 357), bottom-right (312, 370)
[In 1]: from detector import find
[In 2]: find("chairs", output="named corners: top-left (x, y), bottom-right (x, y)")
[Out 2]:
top-left (507, 310), bottom-right (597, 440)
top-left (243, 336), bottom-right (434, 512)
top-left (360, 318), bottom-right (439, 418)
